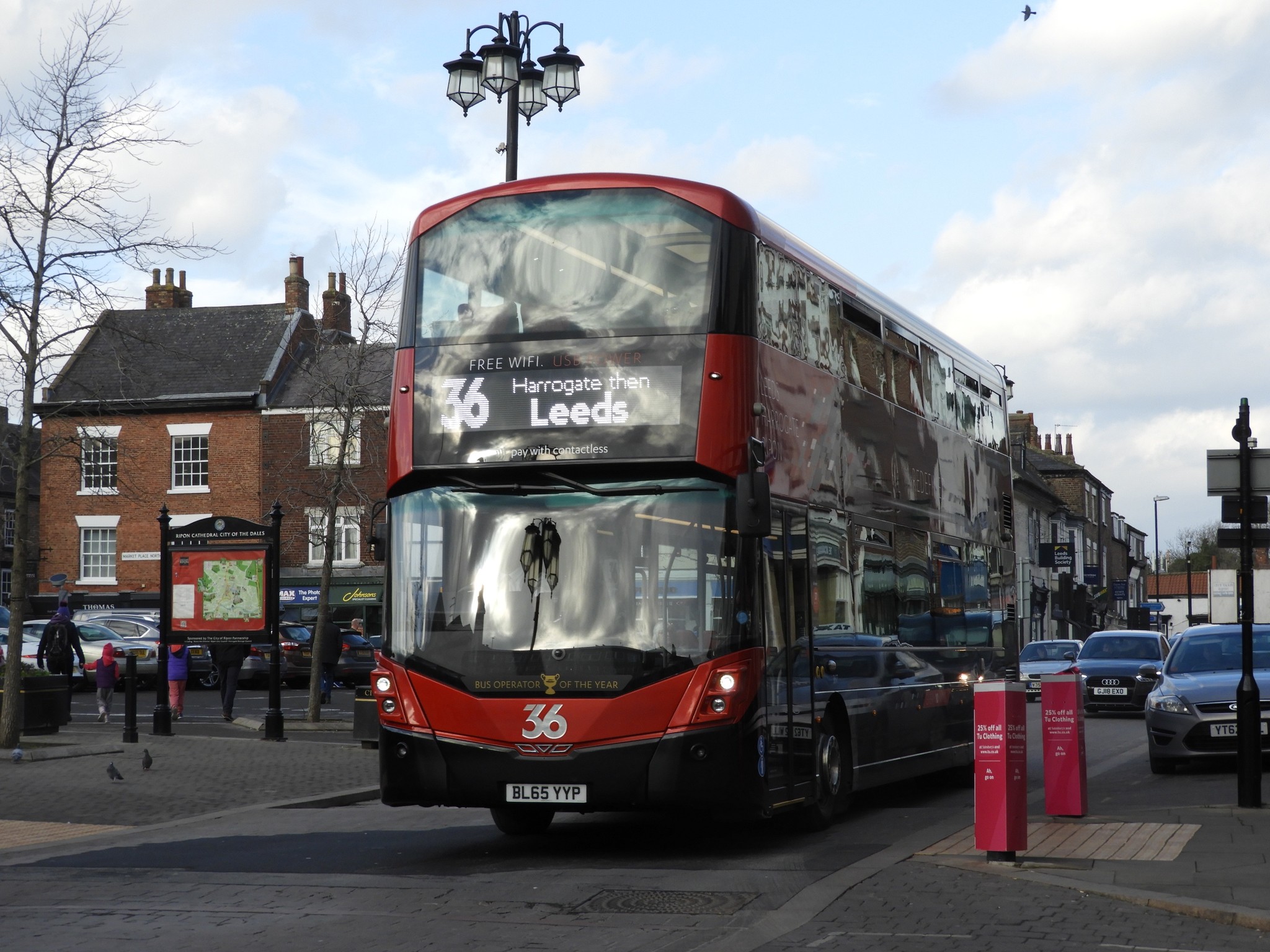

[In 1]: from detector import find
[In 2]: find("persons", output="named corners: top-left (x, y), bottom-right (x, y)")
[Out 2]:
top-left (80, 643), bottom-right (120, 723)
top-left (164, 640), bottom-right (193, 720)
top-left (36, 602), bottom-right (86, 722)
top-left (204, 641), bottom-right (253, 722)
top-left (309, 612), bottom-right (362, 705)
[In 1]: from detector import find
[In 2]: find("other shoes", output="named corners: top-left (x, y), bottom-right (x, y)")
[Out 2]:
top-left (322, 692), bottom-right (327, 704)
top-left (172, 707), bottom-right (182, 720)
top-left (97, 710), bottom-right (106, 721)
top-left (327, 694), bottom-right (331, 703)
top-left (105, 716), bottom-right (111, 722)
top-left (221, 711), bottom-right (233, 721)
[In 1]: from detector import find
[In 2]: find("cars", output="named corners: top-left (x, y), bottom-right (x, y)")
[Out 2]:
top-left (0, 604), bottom-right (384, 691)
top-left (1063, 629), bottom-right (1172, 714)
top-left (1137, 624), bottom-right (1270, 775)
top-left (1167, 632), bottom-right (1183, 648)
top-left (1018, 639), bottom-right (1085, 701)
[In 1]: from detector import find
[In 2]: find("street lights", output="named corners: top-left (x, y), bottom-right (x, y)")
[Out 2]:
top-left (1152, 495), bottom-right (1171, 632)
top-left (517, 516), bottom-right (565, 651)
top-left (441, 9), bottom-right (585, 183)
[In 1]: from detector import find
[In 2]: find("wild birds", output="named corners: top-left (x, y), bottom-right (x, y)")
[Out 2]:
top-left (1021, 5), bottom-right (1036, 21)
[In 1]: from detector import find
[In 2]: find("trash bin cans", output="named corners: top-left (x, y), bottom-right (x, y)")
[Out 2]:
top-left (354, 686), bottom-right (381, 750)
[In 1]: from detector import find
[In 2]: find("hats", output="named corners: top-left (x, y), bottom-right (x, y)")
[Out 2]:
top-left (57, 600), bottom-right (71, 620)
top-left (103, 643), bottom-right (114, 657)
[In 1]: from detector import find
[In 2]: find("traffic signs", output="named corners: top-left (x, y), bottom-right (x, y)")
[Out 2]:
top-left (1138, 602), bottom-right (1165, 612)
top-left (1150, 616), bottom-right (1157, 622)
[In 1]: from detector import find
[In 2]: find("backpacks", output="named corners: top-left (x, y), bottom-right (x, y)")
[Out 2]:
top-left (45, 620), bottom-right (69, 659)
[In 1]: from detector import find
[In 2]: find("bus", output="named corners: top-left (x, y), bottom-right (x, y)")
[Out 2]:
top-left (367, 170), bottom-right (1021, 840)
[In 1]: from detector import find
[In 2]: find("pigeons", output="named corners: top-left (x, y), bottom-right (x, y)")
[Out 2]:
top-left (142, 749), bottom-right (153, 770)
top-left (107, 762), bottom-right (124, 782)
top-left (12, 743), bottom-right (22, 764)
